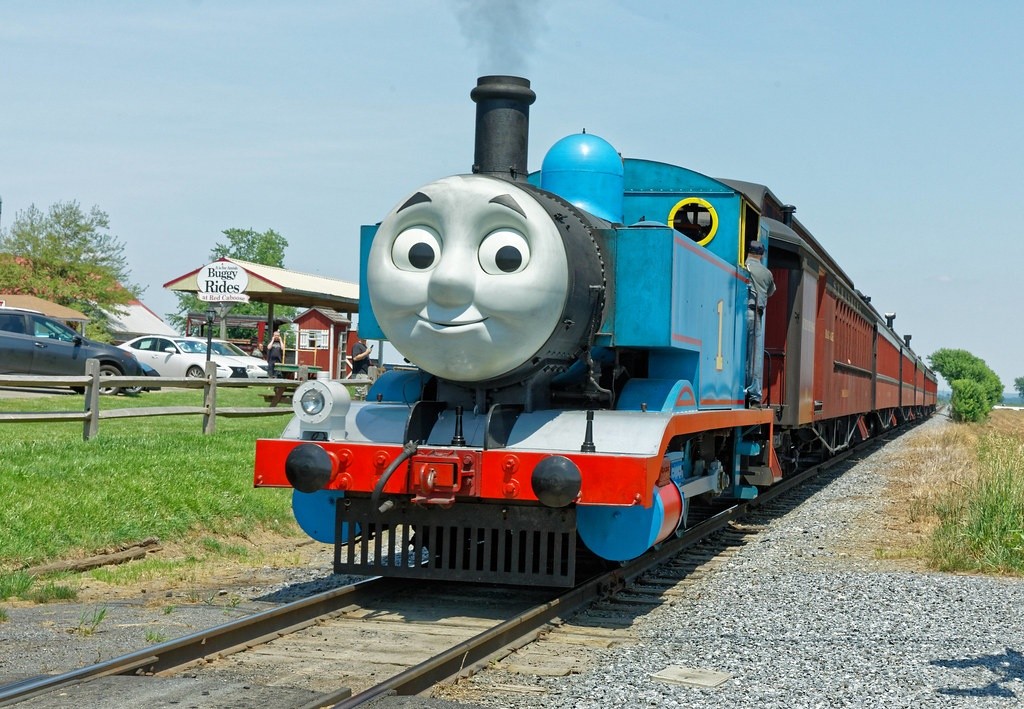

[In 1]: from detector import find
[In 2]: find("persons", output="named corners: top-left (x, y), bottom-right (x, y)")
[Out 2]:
top-left (745, 240), bottom-right (776, 409)
top-left (267, 331), bottom-right (283, 390)
top-left (351, 339), bottom-right (372, 401)
top-left (252, 343), bottom-right (264, 357)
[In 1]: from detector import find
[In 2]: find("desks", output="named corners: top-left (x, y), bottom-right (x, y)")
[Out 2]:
top-left (273, 363), bottom-right (322, 379)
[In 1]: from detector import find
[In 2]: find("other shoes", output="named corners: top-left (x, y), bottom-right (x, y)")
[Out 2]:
top-left (751, 400), bottom-right (761, 409)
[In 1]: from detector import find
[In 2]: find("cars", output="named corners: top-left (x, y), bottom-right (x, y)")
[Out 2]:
top-left (0, 307), bottom-right (142, 398)
top-left (174, 338), bottom-right (282, 378)
top-left (33, 332), bottom-right (162, 396)
top-left (113, 336), bottom-right (255, 390)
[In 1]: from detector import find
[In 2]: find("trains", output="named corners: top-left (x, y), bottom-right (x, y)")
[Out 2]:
top-left (252, 75), bottom-right (938, 591)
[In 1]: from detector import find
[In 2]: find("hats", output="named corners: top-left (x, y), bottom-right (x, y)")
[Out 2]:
top-left (748, 240), bottom-right (765, 255)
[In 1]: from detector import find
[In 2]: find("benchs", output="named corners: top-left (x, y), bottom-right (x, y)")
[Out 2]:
top-left (258, 386), bottom-right (296, 407)
